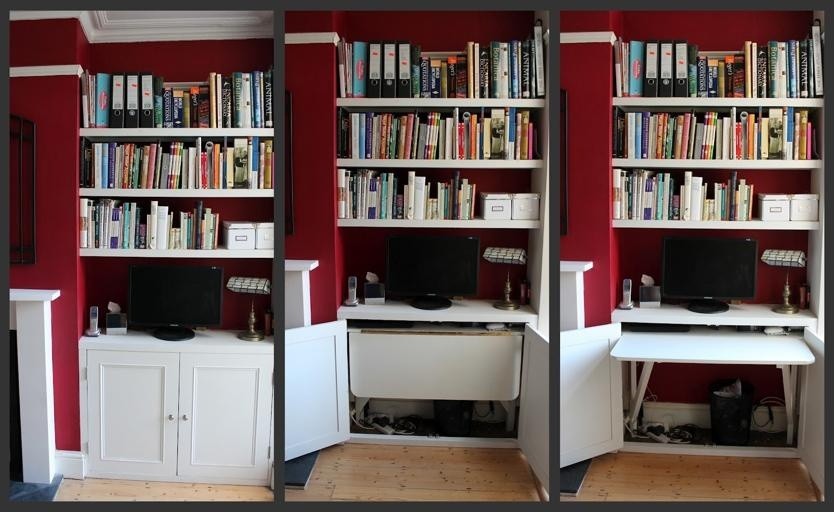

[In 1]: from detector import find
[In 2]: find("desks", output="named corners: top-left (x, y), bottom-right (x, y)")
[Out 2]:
top-left (610, 330), bottom-right (816, 447)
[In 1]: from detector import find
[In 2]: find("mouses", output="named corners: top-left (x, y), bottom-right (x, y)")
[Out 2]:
top-left (764, 327), bottom-right (784, 334)
top-left (485, 323), bottom-right (505, 330)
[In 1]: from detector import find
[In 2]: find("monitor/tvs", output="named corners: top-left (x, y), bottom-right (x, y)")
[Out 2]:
top-left (661, 235), bottom-right (759, 314)
top-left (384, 234), bottom-right (481, 310)
top-left (127, 263), bottom-right (224, 341)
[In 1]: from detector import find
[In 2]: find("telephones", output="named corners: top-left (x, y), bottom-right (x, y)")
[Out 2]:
top-left (83, 305), bottom-right (102, 338)
top-left (617, 277), bottom-right (636, 311)
top-left (343, 275), bottom-right (360, 307)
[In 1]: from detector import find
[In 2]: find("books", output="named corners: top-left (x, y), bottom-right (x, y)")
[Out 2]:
top-left (613, 24), bottom-right (825, 98)
top-left (79, 66), bottom-right (273, 252)
top-left (338, 169), bottom-right (476, 221)
top-left (612, 105), bottom-right (811, 160)
top-left (337, 25), bottom-right (545, 99)
top-left (338, 106), bottom-right (537, 160)
top-left (612, 168), bottom-right (754, 220)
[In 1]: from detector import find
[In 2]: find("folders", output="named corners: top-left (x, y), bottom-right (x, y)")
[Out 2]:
top-left (108, 70), bottom-right (157, 128)
top-left (366, 41), bottom-right (413, 99)
top-left (642, 40), bottom-right (690, 96)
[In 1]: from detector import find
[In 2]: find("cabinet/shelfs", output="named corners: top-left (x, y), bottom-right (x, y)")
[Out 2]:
top-left (612, 97), bottom-right (822, 232)
top-left (76, 126), bottom-right (275, 260)
top-left (79, 327), bottom-right (274, 488)
top-left (336, 97), bottom-right (541, 230)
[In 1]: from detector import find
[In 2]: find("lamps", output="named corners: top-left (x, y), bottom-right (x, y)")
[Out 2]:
top-left (225, 276), bottom-right (271, 341)
top-left (483, 244), bottom-right (526, 312)
top-left (760, 248), bottom-right (808, 317)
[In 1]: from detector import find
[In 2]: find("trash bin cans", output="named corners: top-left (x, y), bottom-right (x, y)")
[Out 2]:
top-left (435, 400), bottom-right (477, 438)
top-left (708, 379), bottom-right (757, 446)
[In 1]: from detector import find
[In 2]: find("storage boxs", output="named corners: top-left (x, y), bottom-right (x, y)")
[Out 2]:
top-left (759, 192), bottom-right (820, 223)
top-left (221, 219), bottom-right (274, 249)
top-left (479, 191), bottom-right (540, 221)
